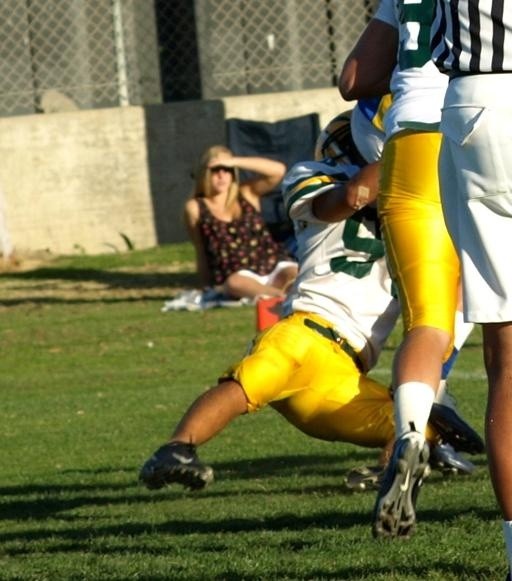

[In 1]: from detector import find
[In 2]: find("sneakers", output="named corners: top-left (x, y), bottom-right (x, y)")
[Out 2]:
top-left (342, 385), bottom-right (485, 540)
top-left (140, 444), bottom-right (215, 489)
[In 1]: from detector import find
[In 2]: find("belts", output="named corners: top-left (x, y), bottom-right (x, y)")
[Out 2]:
top-left (305, 319), bottom-right (366, 372)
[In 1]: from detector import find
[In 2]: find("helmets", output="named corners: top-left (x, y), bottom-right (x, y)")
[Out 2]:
top-left (315, 111), bottom-right (368, 168)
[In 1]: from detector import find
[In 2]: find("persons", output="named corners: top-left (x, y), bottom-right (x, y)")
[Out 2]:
top-left (339, 2), bottom-right (511, 540)
top-left (137, 108), bottom-right (475, 493)
top-left (183, 144), bottom-right (300, 298)
